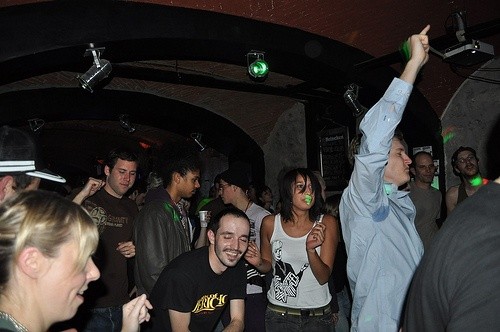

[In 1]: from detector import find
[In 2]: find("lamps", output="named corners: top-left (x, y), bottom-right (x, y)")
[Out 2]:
top-left (246, 50), bottom-right (268, 79)
top-left (120, 114), bottom-right (136, 133)
top-left (189, 132), bottom-right (207, 152)
top-left (76, 44), bottom-right (112, 94)
top-left (344, 83), bottom-right (369, 135)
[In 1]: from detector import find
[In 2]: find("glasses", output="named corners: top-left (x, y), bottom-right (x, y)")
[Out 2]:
top-left (415, 163), bottom-right (435, 170)
top-left (456, 156), bottom-right (476, 164)
top-left (219, 183), bottom-right (228, 189)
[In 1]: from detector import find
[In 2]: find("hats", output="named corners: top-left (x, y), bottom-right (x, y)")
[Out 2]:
top-left (0, 124), bottom-right (67, 183)
top-left (220, 168), bottom-right (248, 188)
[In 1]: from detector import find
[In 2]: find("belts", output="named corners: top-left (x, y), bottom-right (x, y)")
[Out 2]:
top-left (267, 302), bottom-right (332, 316)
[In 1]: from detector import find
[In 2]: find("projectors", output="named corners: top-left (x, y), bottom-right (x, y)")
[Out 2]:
top-left (443, 40), bottom-right (494, 68)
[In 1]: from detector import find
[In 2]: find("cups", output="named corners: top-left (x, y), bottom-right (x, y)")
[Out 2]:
top-left (199, 211), bottom-right (208, 227)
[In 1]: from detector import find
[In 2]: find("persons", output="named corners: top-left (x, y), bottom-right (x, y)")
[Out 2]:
top-left (337, 23), bottom-right (432, 331)
top-left (445, 146), bottom-right (493, 216)
top-left (400, 177), bottom-right (499, 331)
top-left (400, 150), bottom-right (442, 245)
top-left (0, 126), bottom-right (41, 204)
top-left (1, 190), bottom-right (154, 331)
top-left (69, 146), bottom-right (351, 331)
